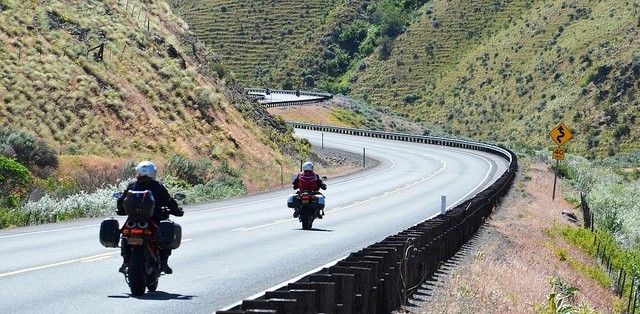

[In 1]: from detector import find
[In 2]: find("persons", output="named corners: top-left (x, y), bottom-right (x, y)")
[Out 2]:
top-left (117, 161), bottom-right (184, 274)
top-left (296, 89), bottom-right (299, 96)
top-left (293, 162), bottom-right (328, 219)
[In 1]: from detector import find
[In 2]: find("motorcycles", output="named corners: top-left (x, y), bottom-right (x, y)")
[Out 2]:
top-left (288, 175), bottom-right (327, 230)
top-left (100, 192), bottom-right (186, 297)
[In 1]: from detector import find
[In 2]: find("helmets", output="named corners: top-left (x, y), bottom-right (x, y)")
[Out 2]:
top-left (303, 162), bottom-right (313, 172)
top-left (137, 161), bottom-right (156, 178)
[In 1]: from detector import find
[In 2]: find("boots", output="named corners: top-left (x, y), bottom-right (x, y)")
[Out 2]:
top-left (161, 254), bottom-right (172, 274)
top-left (119, 255), bottom-right (129, 273)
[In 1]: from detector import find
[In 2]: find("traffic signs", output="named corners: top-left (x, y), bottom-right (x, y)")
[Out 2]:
top-left (552, 147), bottom-right (565, 160)
top-left (549, 123), bottom-right (573, 147)
